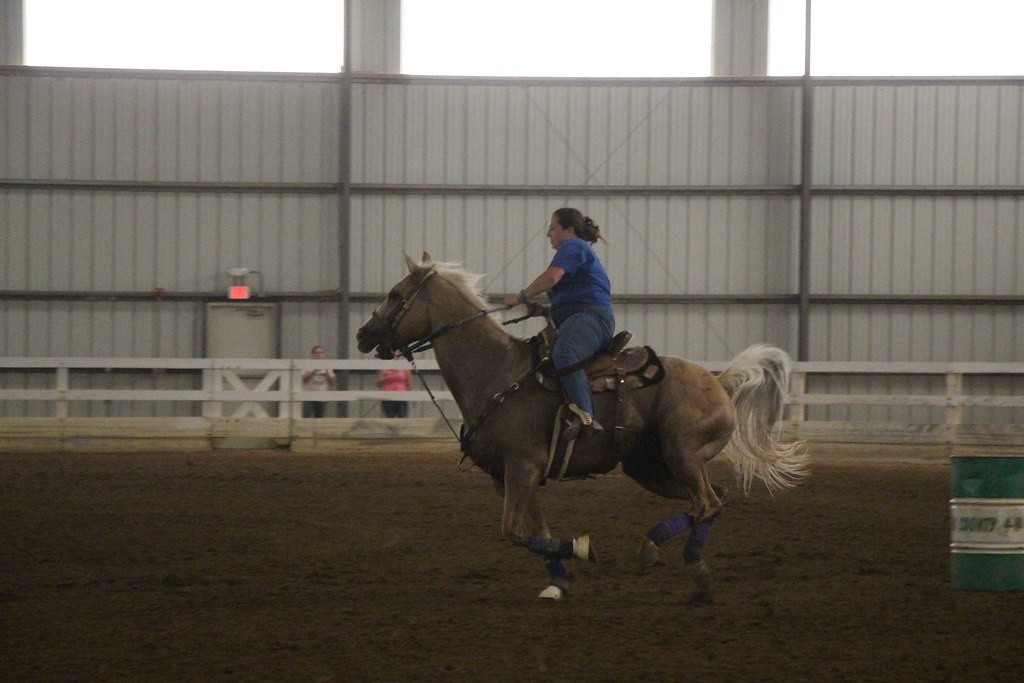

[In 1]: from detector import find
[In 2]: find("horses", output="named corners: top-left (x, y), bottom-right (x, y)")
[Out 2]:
top-left (355, 248), bottom-right (811, 601)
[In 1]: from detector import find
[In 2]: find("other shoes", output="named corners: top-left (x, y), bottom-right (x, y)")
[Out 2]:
top-left (562, 418), bottom-right (603, 442)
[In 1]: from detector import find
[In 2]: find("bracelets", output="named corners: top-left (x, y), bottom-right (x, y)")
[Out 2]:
top-left (518, 289), bottom-right (527, 303)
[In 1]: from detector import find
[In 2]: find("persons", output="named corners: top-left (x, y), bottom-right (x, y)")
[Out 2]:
top-left (301, 346), bottom-right (335, 419)
top-left (375, 350), bottom-right (419, 419)
top-left (503, 208), bottom-right (616, 438)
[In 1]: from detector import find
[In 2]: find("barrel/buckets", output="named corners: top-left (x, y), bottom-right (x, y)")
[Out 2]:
top-left (948, 456), bottom-right (1024, 592)
